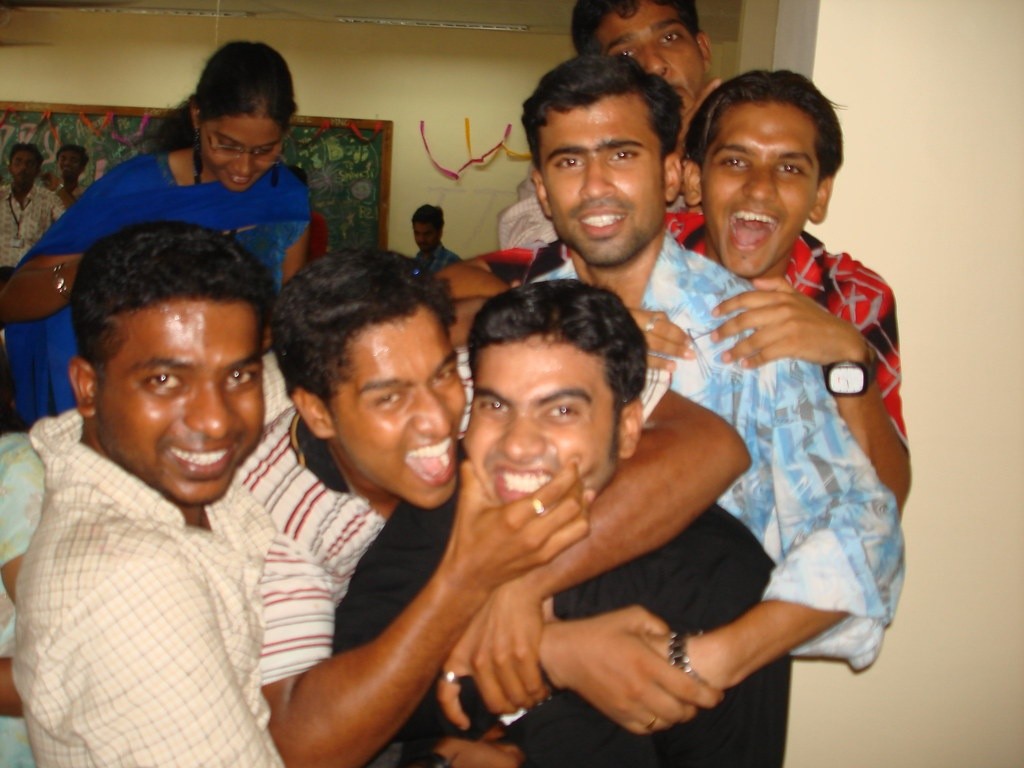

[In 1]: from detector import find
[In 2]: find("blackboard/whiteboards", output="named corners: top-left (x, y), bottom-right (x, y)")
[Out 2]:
top-left (0, 97), bottom-right (396, 302)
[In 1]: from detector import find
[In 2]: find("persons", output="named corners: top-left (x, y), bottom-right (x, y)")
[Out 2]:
top-left (0, 0), bottom-right (913, 768)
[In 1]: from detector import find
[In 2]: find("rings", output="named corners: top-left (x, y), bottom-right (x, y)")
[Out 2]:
top-left (645, 317), bottom-right (660, 332)
top-left (438, 670), bottom-right (459, 684)
top-left (646, 715), bottom-right (658, 730)
top-left (528, 495), bottom-right (545, 515)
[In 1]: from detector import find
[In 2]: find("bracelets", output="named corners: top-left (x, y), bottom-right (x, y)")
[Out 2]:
top-left (668, 627), bottom-right (713, 687)
top-left (52, 262), bottom-right (72, 300)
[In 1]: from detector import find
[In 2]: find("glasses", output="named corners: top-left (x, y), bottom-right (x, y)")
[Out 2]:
top-left (200, 109), bottom-right (285, 164)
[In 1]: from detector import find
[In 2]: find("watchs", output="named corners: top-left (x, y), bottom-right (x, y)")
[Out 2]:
top-left (821, 336), bottom-right (878, 397)
